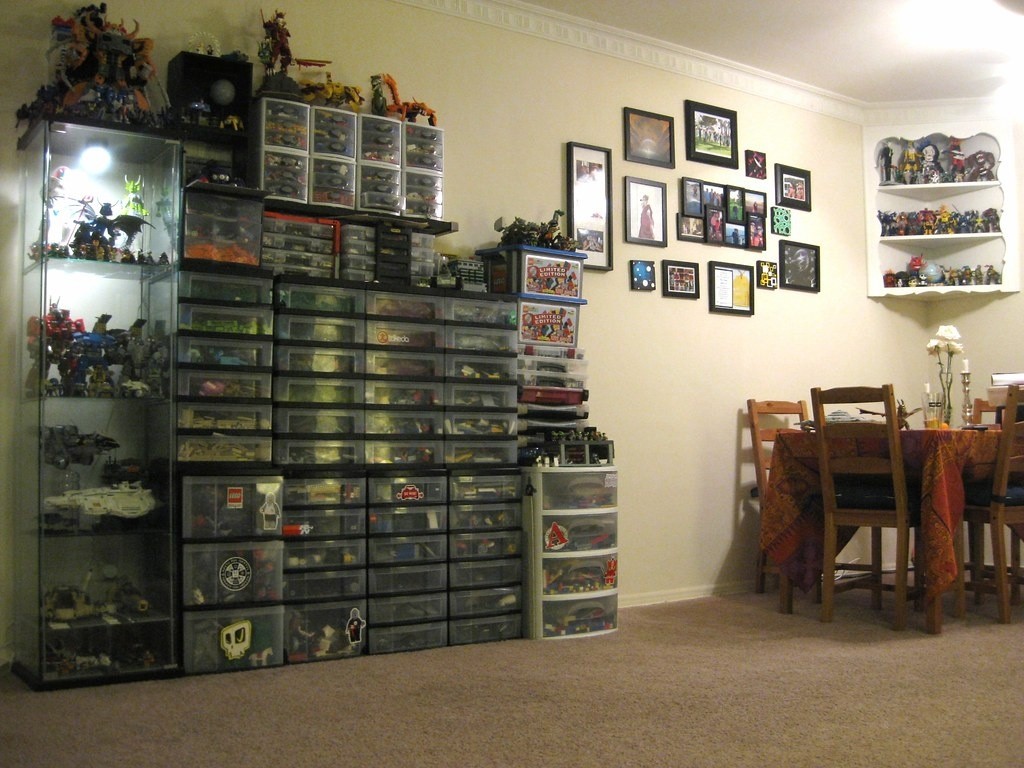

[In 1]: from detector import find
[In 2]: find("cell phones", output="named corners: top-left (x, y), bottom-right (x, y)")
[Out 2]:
top-left (962, 426), bottom-right (989, 430)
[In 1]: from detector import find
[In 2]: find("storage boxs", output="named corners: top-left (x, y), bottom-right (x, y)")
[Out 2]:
top-left (174, 95), bottom-right (622, 675)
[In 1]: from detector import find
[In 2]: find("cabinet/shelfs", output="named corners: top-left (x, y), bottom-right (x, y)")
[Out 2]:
top-left (861, 122), bottom-right (1022, 297)
top-left (10, 112), bottom-right (180, 694)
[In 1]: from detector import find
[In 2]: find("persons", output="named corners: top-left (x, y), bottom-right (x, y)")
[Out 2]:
top-left (752, 202), bottom-right (759, 212)
top-left (690, 187), bottom-right (698, 200)
top-left (639, 195), bottom-right (654, 240)
top-left (877, 205), bottom-right (1001, 236)
top-left (47, 314), bottom-right (168, 399)
top-left (16, 1), bottom-right (248, 134)
top-left (260, 8), bottom-right (293, 75)
top-left (785, 181), bottom-right (804, 199)
top-left (582, 235), bottom-right (603, 252)
top-left (710, 212), bottom-right (721, 238)
top-left (731, 228), bottom-right (743, 245)
top-left (882, 140), bottom-right (995, 183)
top-left (882, 254), bottom-right (1002, 288)
top-left (704, 188), bottom-right (722, 206)
top-left (697, 121), bottom-right (731, 146)
top-left (25, 297), bottom-right (84, 397)
top-left (751, 221), bottom-right (763, 246)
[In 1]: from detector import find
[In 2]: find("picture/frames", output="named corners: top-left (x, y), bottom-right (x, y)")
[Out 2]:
top-left (566, 97), bottom-right (820, 319)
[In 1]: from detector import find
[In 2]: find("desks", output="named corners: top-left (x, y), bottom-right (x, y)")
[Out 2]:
top-left (759, 425), bottom-right (1024, 635)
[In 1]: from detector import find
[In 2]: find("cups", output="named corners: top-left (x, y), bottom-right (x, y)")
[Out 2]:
top-left (922, 382), bottom-right (944, 428)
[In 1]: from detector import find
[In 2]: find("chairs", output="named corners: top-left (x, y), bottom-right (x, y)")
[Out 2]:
top-left (808, 381), bottom-right (925, 632)
top-left (746, 394), bottom-right (822, 604)
top-left (960, 384), bottom-right (1024, 626)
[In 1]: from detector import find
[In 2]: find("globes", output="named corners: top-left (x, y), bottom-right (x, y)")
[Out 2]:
top-left (918, 260), bottom-right (947, 287)
